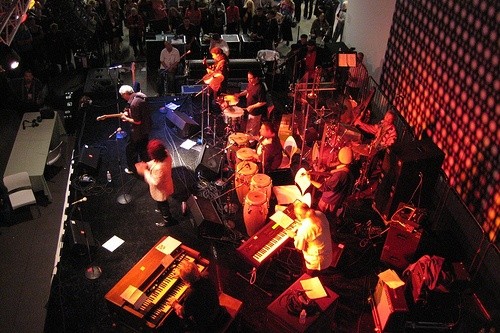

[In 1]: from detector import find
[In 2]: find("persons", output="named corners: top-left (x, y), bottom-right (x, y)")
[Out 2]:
top-left (173, 262), bottom-right (222, 329)
top-left (285, 203), bottom-right (332, 278)
top-left (346, 52), bottom-right (368, 103)
top-left (308, 146), bottom-right (363, 226)
top-left (354, 110), bottom-right (398, 155)
top-left (16, 70), bottom-right (44, 118)
top-left (25, 0), bottom-right (348, 85)
top-left (233, 70), bottom-right (268, 137)
top-left (155, 41), bottom-right (181, 97)
top-left (118, 85), bottom-right (152, 173)
top-left (205, 47), bottom-right (230, 109)
top-left (240, 120), bottom-right (283, 171)
top-left (135, 139), bottom-right (173, 228)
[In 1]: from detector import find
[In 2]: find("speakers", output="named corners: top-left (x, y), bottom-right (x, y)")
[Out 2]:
top-left (66, 220), bottom-right (95, 251)
top-left (166, 109), bottom-right (199, 139)
top-left (194, 142), bottom-right (225, 180)
top-left (78, 144), bottom-right (101, 173)
top-left (186, 194), bottom-right (225, 236)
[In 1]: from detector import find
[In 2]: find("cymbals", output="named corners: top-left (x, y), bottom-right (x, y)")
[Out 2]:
top-left (358, 144), bottom-right (377, 156)
top-left (340, 121), bottom-right (360, 134)
top-left (203, 72), bottom-right (224, 85)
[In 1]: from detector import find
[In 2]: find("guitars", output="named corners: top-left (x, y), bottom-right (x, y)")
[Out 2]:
top-left (96, 108), bottom-right (132, 121)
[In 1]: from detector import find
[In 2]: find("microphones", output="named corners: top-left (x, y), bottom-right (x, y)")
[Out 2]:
top-left (109, 127), bottom-right (122, 139)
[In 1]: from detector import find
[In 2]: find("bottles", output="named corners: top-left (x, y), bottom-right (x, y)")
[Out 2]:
top-left (197, 136), bottom-right (202, 145)
top-left (107, 171), bottom-right (111, 182)
top-left (299, 309), bottom-right (306, 324)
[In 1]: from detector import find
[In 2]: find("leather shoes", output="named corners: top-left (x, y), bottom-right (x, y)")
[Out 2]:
top-left (154, 208), bottom-right (170, 228)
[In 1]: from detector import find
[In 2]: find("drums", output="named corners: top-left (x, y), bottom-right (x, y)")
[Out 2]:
top-left (312, 128), bottom-right (360, 171)
top-left (220, 94), bottom-right (272, 236)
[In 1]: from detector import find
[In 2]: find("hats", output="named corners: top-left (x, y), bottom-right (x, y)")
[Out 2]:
top-left (338, 147), bottom-right (353, 165)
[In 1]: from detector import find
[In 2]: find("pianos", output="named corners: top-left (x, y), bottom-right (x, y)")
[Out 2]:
top-left (236, 199), bottom-right (303, 296)
top-left (104, 235), bottom-right (209, 333)
